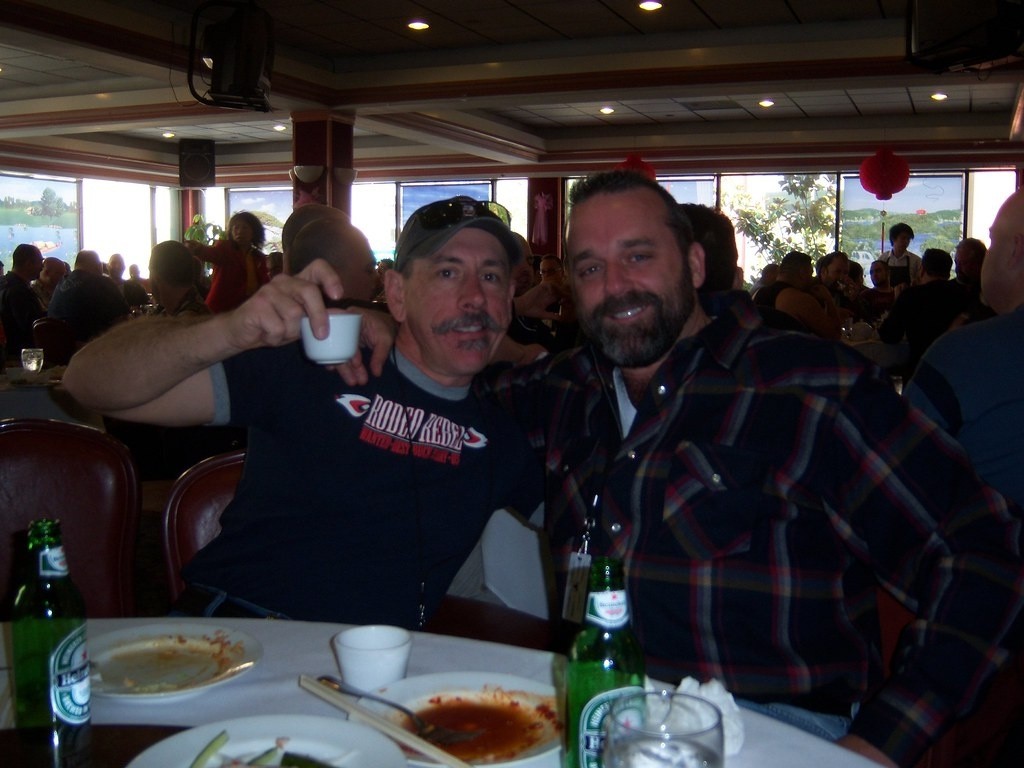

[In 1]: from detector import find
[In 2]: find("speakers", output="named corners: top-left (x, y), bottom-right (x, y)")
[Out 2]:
top-left (178, 138), bottom-right (218, 188)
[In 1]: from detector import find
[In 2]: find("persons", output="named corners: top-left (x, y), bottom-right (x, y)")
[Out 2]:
top-left (485, 170), bottom-right (1024, 768)
top-left (0, 196), bottom-right (577, 632)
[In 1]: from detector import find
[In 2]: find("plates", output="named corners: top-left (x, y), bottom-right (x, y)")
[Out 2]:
top-left (88, 621), bottom-right (265, 707)
top-left (124, 711), bottom-right (409, 767)
top-left (345, 671), bottom-right (560, 767)
top-left (7, 379), bottom-right (62, 387)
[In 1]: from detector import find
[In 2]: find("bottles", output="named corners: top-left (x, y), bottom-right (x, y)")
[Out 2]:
top-left (10, 516), bottom-right (90, 767)
top-left (552, 525), bottom-right (648, 768)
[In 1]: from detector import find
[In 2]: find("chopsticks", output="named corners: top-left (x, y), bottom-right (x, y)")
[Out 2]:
top-left (298, 673), bottom-right (469, 767)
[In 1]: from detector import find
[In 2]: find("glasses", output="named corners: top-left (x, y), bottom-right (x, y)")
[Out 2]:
top-left (540, 267), bottom-right (561, 275)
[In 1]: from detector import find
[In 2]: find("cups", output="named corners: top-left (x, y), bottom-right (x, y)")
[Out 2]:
top-left (300, 314), bottom-right (362, 364)
top-left (333, 623), bottom-right (414, 691)
top-left (891, 375), bottom-right (903, 395)
top-left (21, 348), bottom-right (44, 373)
top-left (602, 688), bottom-right (725, 768)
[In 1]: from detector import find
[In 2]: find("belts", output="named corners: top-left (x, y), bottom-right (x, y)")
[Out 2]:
top-left (732, 688), bottom-right (860, 718)
top-left (172, 585), bottom-right (271, 619)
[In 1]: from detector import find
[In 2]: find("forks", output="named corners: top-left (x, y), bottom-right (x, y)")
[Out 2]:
top-left (316, 675), bottom-right (486, 743)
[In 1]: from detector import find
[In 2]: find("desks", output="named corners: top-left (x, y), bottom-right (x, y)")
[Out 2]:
top-left (0, 618), bottom-right (894, 768)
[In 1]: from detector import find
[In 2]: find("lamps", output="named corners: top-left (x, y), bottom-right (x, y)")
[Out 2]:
top-left (335, 167), bottom-right (358, 185)
top-left (293, 165), bottom-right (323, 183)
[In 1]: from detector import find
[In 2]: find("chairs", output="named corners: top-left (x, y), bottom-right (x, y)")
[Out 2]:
top-left (161, 447), bottom-right (250, 602)
top-left (0, 420), bottom-right (142, 617)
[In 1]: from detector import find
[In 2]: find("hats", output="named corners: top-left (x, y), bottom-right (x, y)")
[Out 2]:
top-left (395, 195), bottom-right (522, 271)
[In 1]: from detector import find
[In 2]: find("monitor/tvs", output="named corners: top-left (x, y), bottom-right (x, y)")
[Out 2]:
top-left (203, 3), bottom-right (275, 97)
top-left (906, 0), bottom-right (1024, 72)
